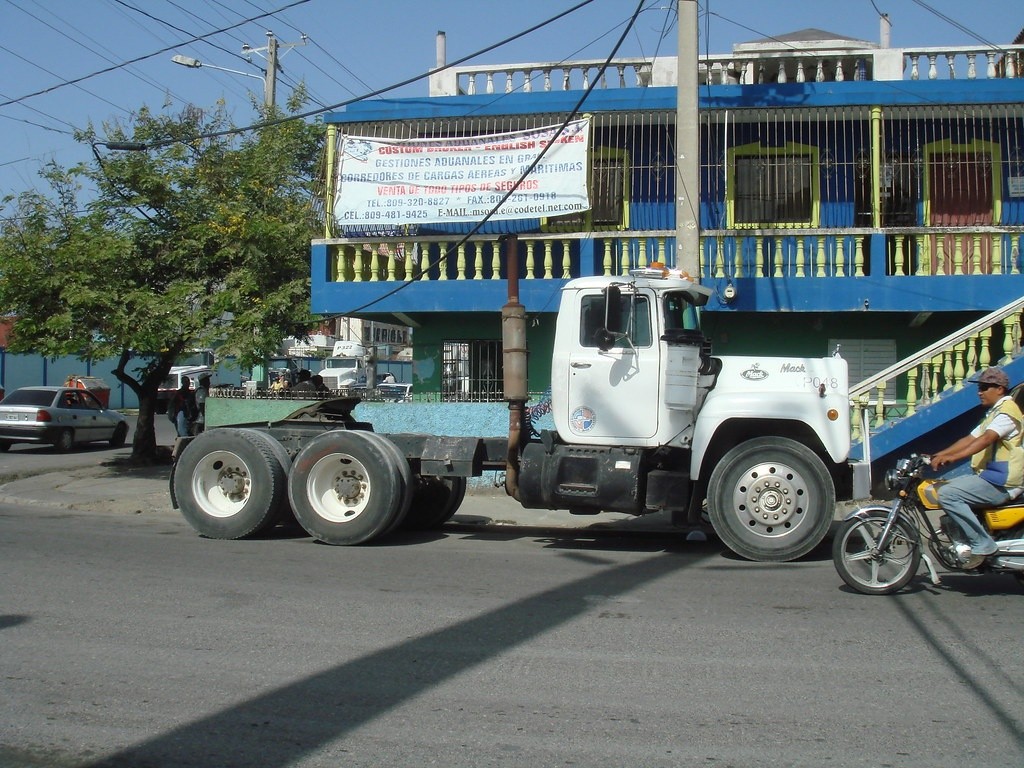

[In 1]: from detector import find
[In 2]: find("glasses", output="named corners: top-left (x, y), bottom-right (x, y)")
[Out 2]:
top-left (977, 386), bottom-right (998, 391)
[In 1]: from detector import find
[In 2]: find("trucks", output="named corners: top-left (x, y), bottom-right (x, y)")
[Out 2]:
top-left (268, 368), bottom-right (300, 390)
top-left (157, 348), bottom-right (234, 415)
top-left (318, 340), bottom-right (368, 395)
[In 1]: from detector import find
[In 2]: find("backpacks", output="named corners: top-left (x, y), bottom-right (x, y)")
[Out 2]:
top-left (186, 387), bottom-right (203, 425)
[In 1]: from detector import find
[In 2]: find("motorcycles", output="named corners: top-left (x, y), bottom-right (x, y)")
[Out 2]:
top-left (832, 453), bottom-right (1024, 596)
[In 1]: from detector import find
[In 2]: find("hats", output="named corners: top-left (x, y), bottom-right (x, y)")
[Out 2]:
top-left (296, 370), bottom-right (311, 377)
top-left (198, 374), bottom-right (212, 380)
top-left (967, 367), bottom-right (1009, 388)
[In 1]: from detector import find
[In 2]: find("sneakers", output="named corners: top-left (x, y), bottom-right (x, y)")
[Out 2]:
top-left (961, 548), bottom-right (999, 570)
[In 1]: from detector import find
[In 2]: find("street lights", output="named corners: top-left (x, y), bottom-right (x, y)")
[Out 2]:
top-left (171, 54), bottom-right (277, 122)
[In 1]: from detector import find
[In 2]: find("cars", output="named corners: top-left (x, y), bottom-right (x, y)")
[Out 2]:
top-left (360, 383), bottom-right (413, 403)
top-left (375, 372), bottom-right (397, 386)
top-left (0, 386), bottom-right (130, 454)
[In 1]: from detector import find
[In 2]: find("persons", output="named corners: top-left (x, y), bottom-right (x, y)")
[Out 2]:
top-left (268, 369), bottom-right (334, 397)
top-left (172, 372), bottom-right (213, 437)
top-left (920, 366), bottom-right (1024, 569)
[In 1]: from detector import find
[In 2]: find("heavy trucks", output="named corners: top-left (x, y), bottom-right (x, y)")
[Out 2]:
top-left (167, 231), bottom-right (852, 562)
